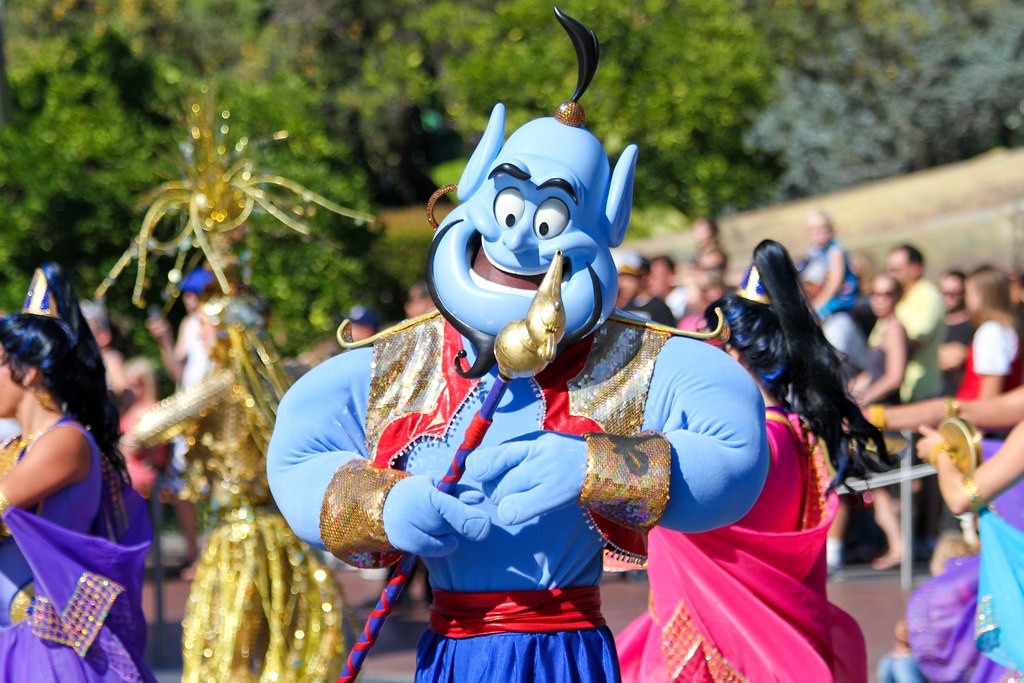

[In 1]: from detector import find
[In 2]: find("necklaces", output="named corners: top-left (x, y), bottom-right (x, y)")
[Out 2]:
top-left (0, 430), bottom-right (42, 477)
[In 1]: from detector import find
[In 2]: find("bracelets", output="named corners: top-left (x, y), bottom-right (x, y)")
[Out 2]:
top-left (870, 402), bottom-right (885, 432)
top-left (928, 444), bottom-right (957, 468)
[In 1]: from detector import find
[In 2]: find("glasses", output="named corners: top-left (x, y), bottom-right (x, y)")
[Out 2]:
top-left (870, 290), bottom-right (894, 298)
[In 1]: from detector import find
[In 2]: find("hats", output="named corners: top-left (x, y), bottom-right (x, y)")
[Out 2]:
top-left (345, 304), bottom-right (381, 332)
top-left (610, 245), bottom-right (650, 278)
top-left (173, 267), bottom-right (220, 296)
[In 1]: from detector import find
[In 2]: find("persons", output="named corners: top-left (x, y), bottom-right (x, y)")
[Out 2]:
top-left (136, 282), bottom-right (355, 682)
top-left (265, 4), bottom-right (771, 683)
top-left (612, 239), bottom-right (887, 683)
top-left (0, 262), bottom-right (163, 683)
top-left (614, 208), bottom-right (1023, 580)
top-left (348, 281), bottom-right (436, 350)
top-left (866, 384), bottom-right (1023, 683)
top-left (81, 269), bottom-right (216, 580)
